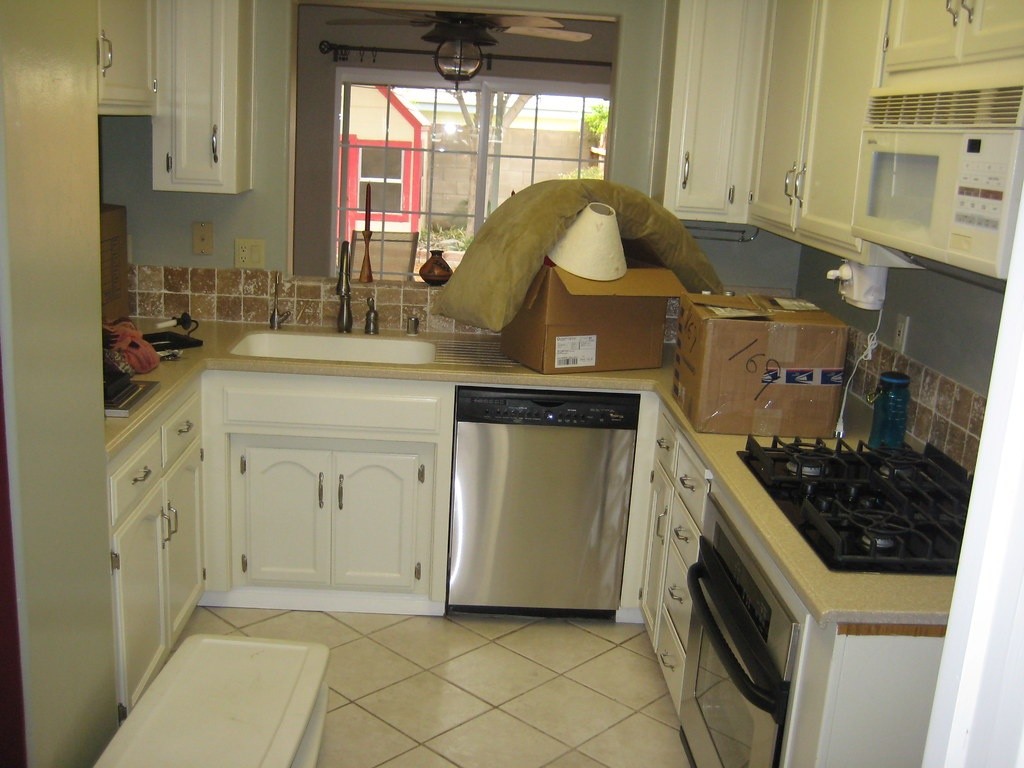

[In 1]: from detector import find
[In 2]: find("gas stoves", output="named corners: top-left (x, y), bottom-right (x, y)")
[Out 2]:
top-left (737, 432), bottom-right (970, 575)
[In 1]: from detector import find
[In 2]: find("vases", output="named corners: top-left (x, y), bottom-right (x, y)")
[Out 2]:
top-left (420, 250), bottom-right (453, 286)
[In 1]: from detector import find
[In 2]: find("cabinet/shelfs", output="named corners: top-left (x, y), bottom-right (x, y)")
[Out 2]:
top-left (638, 411), bottom-right (711, 717)
top-left (650, 0), bottom-right (1024, 269)
top-left (106, 369), bottom-right (455, 726)
top-left (98, 0), bottom-right (257, 194)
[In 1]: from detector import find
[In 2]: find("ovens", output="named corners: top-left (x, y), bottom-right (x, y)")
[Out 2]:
top-left (678, 493), bottom-right (947, 768)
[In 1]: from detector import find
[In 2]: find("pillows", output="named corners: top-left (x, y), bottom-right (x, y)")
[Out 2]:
top-left (427, 177), bottom-right (724, 332)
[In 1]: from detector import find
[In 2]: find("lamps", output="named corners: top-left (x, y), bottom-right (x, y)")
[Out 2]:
top-left (365, 183), bottom-right (371, 231)
top-left (422, 10), bottom-right (499, 92)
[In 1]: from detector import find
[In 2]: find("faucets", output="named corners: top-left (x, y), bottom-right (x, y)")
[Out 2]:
top-left (336, 240), bottom-right (353, 333)
top-left (269, 270), bottom-right (291, 330)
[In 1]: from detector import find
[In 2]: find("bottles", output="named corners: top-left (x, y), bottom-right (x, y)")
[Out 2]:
top-left (724, 292), bottom-right (735, 297)
top-left (867, 371), bottom-right (911, 449)
top-left (407, 317), bottom-right (419, 334)
top-left (365, 298), bottom-right (379, 334)
top-left (702, 291), bottom-right (711, 295)
top-left (419, 250), bottom-right (453, 286)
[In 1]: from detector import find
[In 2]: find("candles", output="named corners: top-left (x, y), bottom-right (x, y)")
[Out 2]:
top-left (155, 319), bottom-right (177, 328)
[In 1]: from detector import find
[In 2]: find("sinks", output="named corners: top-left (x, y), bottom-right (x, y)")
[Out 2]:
top-left (227, 328), bottom-right (436, 365)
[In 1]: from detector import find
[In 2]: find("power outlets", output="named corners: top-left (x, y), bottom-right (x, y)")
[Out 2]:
top-left (234, 239), bottom-right (265, 270)
top-left (893, 313), bottom-right (909, 355)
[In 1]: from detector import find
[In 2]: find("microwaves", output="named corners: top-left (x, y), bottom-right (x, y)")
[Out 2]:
top-left (852, 126), bottom-right (1024, 280)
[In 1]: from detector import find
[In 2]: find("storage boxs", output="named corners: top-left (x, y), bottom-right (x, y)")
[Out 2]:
top-left (500, 256), bottom-right (689, 375)
top-left (100, 203), bottom-right (129, 324)
top-left (671, 293), bottom-right (850, 439)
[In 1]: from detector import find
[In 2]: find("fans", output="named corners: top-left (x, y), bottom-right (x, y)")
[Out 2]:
top-left (324, 6), bottom-right (592, 42)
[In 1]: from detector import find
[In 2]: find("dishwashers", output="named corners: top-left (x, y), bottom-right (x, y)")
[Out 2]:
top-left (448, 387), bottom-right (641, 619)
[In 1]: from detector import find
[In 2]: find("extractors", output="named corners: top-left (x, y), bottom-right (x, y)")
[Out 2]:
top-left (876, 243), bottom-right (1007, 293)
